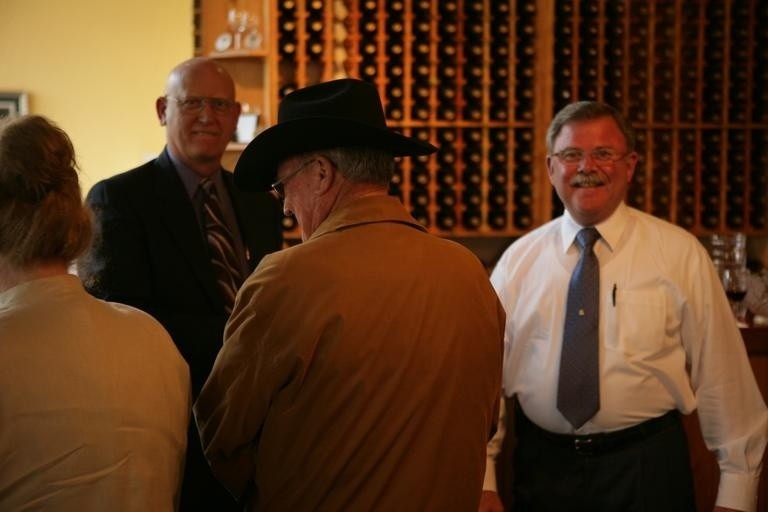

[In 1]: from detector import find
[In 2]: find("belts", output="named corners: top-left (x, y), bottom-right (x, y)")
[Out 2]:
top-left (512, 406), bottom-right (680, 457)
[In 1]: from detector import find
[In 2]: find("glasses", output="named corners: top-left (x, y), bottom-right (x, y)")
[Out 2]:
top-left (272, 153), bottom-right (337, 199)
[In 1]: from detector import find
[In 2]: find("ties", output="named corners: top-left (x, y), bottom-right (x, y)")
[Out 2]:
top-left (198, 177), bottom-right (253, 318)
top-left (556, 226), bottom-right (602, 429)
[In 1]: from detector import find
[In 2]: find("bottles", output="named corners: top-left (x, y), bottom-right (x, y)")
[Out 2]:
top-left (711, 232), bottom-right (747, 282)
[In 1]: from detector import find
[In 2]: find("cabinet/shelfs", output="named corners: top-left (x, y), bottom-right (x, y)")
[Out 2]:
top-left (200, 46), bottom-right (273, 156)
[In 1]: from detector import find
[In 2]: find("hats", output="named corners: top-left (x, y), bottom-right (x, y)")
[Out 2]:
top-left (232, 78), bottom-right (438, 193)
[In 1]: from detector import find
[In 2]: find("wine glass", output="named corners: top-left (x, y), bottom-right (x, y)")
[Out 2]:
top-left (723, 269), bottom-right (750, 328)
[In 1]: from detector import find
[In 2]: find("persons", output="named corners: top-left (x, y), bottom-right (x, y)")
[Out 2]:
top-left (79, 54), bottom-right (285, 512)
top-left (0, 112), bottom-right (195, 512)
top-left (194, 76), bottom-right (509, 511)
top-left (488, 98), bottom-right (768, 512)
